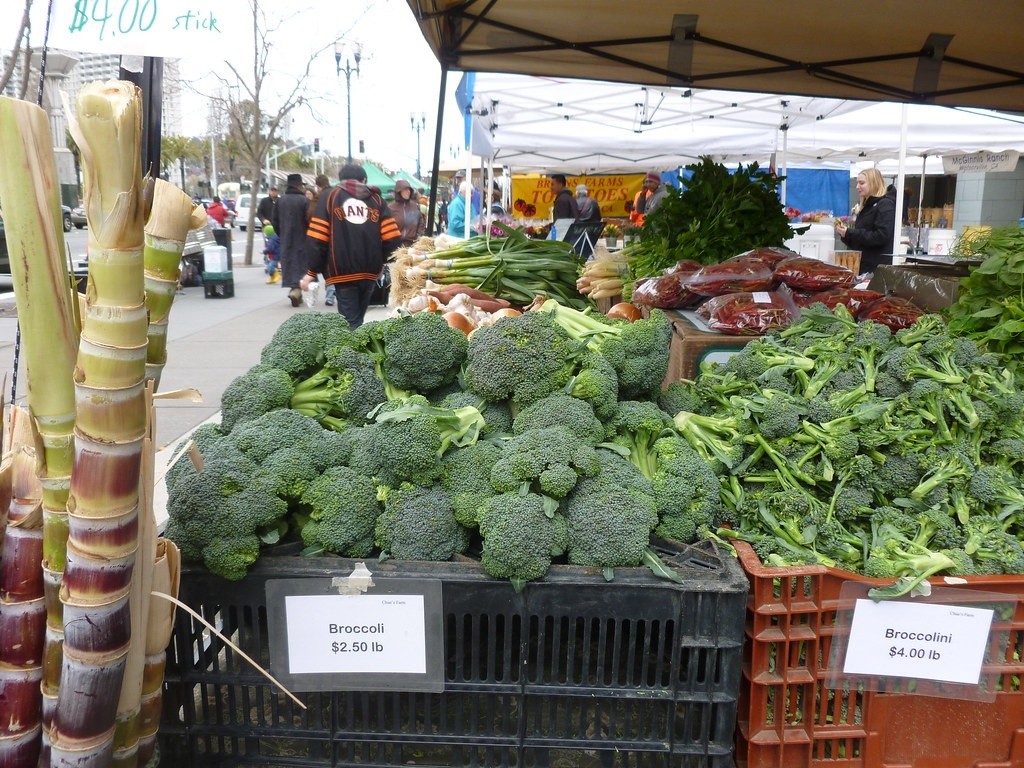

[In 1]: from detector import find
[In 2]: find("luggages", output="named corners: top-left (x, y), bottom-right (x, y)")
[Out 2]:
top-left (368, 265), bottom-right (391, 308)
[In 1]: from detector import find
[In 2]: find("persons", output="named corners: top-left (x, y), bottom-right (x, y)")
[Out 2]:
top-left (836, 168), bottom-right (897, 277)
top-left (258, 165), bottom-right (427, 333)
top-left (624, 170), bottom-right (663, 227)
top-left (440, 169), bottom-right (511, 240)
top-left (190, 189), bottom-right (238, 229)
top-left (538, 174), bottom-right (603, 259)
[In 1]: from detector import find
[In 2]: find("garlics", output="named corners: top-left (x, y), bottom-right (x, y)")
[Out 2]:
top-left (396, 293), bottom-right (492, 330)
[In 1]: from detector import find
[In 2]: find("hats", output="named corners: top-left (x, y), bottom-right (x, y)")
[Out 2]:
top-left (213, 197), bottom-right (221, 202)
top-left (264, 224), bottom-right (274, 236)
top-left (285, 174), bottom-right (308, 185)
top-left (576, 185), bottom-right (587, 194)
top-left (455, 169), bottom-right (466, 178)
top-left (645, 173), bottom-right (661, 184)
top-left (270, 184), bottom-right (279, 190)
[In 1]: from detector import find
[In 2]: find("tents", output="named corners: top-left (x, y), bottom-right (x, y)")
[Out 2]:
top-left (452, 68), bottom-right (881, 254)
top-left (406, 0), bottom-right (1024, 238)
top-left (330, 163), bottom-right (441, 199)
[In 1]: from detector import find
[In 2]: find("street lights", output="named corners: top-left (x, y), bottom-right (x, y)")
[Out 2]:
top-left (334, 38), bottom-right (362, 164)
top-left (449, 145), bottom-right (460, 193)
top-left (410, 110), bottom-right (427, 181)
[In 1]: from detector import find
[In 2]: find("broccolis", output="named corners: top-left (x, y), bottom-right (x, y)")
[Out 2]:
top-left (381, 311), bottom-right (470, 395)
top-left (349, 321), bottom-right (417, 401)
top-left (536, 299), bottom-right (673, 398)
top-left (462, 313), bottom-right (583, 403)
top-left (259, 312), bottom-right (349, 373)
top-left (658, 303), bottom-right (1023, 598)
top-left (164, 329), bottom-right (722, 593)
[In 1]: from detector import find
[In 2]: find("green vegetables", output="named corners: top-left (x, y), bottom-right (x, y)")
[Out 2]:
top-left (618, 152), bottom-right (815, 300)
top-left (943, 213), bottom-right (1024, 388)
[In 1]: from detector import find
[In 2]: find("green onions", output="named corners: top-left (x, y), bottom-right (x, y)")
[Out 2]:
top-left (387, 220), bottom-right (597, 310)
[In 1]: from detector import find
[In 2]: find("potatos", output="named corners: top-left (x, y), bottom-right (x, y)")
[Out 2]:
top-left (426, 291), bottom-right (501, 313)
top-left (496, 299), bottom-right (511, 307)
top-left (439, 284), bottom-right (503, 305)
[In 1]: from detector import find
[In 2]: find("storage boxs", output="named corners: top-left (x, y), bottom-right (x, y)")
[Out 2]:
top-left (159, 208), bottom-right (1024, 768)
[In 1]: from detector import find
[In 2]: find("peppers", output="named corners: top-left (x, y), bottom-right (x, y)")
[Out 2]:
top-left (631, 245), bottom-right (928, 335)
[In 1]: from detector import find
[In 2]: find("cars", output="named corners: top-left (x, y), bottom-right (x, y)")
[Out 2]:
top-left (70, 202), bottom-right (88, 230)
top-left (0, 203), bottom-right (73, 239)
top-left (191, 181), bottom-right (282, 231)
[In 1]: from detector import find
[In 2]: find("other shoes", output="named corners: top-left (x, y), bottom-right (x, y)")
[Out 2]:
top-left (288, 288), bottom-right (301, 307)
top-left (274, 271), bottom-right (282, 283)
top-left (231, 235), bottom-right (236, 241)
top-left (325, 299), bottom-right (334, 306)
top-left (266, 276), bottom-right (274, 284)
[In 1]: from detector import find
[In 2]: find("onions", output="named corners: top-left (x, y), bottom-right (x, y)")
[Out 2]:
top-left (441, 312), bottom-right (476, 336)
top-left (606, 302), bottom-right (645, 321)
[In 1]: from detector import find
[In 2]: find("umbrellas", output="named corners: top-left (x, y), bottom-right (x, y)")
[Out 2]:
top-left (761, 102), bottom-right (1024, 261)
